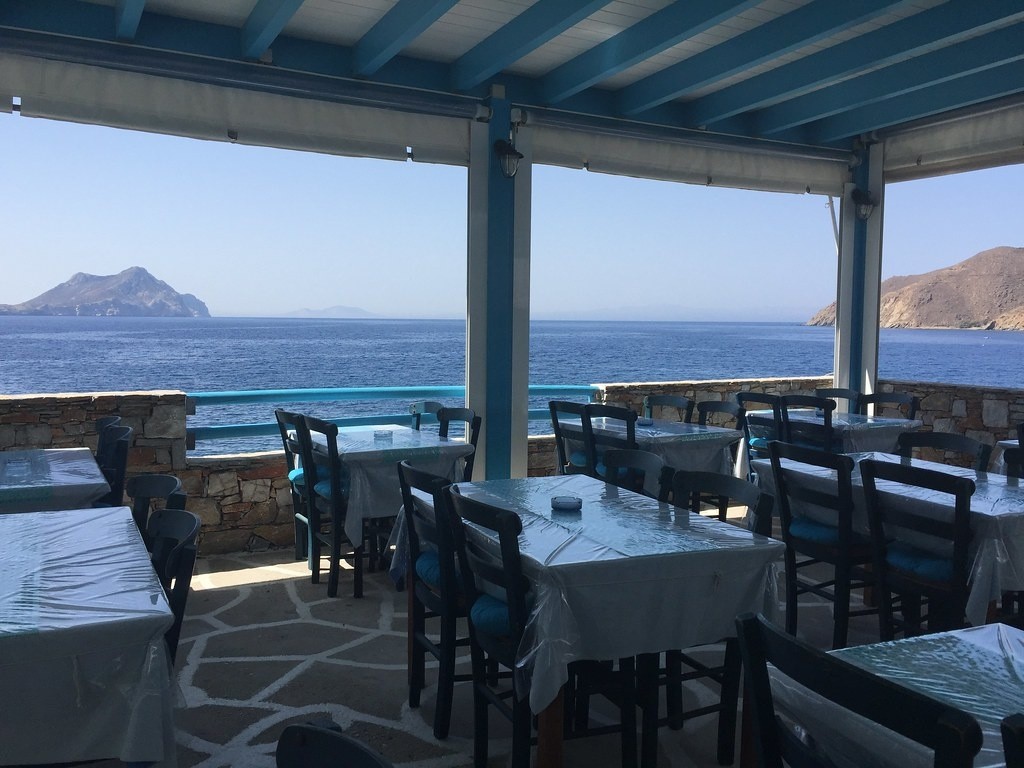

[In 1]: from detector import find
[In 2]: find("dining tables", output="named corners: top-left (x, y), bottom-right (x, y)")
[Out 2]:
top-left (749, 450), bottom-right (1024, 636)
top-left (744, 409), bottom-right (924, 453)
top-left (765, 622), bottom-right (1024, 768)
top-left (549, 416), bottom-right (746, 511)
top-left (0, 505), bottom-right (186, 768)
top-left (285, 423), bottom-right (476, 573)
top-left (0, 447), bottom-right (111, 515)
top-left (399, 472), bottom-right (786, 768)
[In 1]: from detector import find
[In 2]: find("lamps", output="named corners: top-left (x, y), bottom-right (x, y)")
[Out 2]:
top-left (493, 140), bottom-right (524, 178)
top-left (851, 188), bottom-right (877, 221)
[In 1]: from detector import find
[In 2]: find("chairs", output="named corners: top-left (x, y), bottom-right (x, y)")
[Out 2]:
top-left (574, 448), bottom-right (686, 732)
top-left (125, 474), bottom-right (186, 565)
top-left (395, 459), bottom-right (578, 741)
top-left (296, 414), bottom-right (405, 597)
top-left (94, 416), bottom-right (121, 466)
top-left (145, 508), bottom-right (200, 667)
top-left (734, 611), bottom-right (983, 767)
top-left (274, 407), bottom-right (377, 571)
top-left (547, 388), bottom-right (1024, 652)
top-left (639, 468), bottom-right (775, 768)
top-left (275, 723), bottom-right (394, 768)
top-left (408, 402), bottom-right (446, 437)
top-left (437, 407), bottom-right (482, 482)
top-left (93, 426), bottom-right (133, 508)
top-left (441, 485), bottom-right (637, 768)
